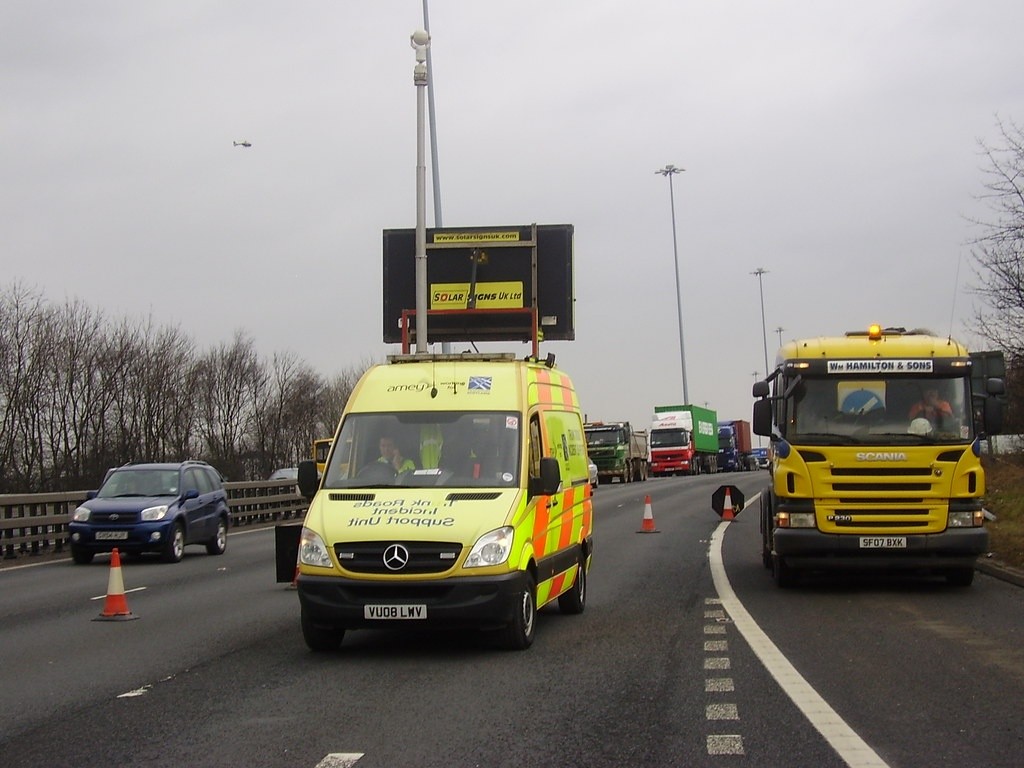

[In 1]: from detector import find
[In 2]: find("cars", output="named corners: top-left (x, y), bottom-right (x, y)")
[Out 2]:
top-left (266, 468), bottom-right (324, 502)
top-left (753, 446), bottom-right (770, 469)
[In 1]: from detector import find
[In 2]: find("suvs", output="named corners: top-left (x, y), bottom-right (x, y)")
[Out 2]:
top-left (68, 460), bottom-right (233, 565)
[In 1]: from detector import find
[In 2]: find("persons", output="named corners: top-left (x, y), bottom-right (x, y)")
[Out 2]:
top-left (910, 379), bottom-right (954, 428)
top-left (377, 433), bottom-right (416, 473)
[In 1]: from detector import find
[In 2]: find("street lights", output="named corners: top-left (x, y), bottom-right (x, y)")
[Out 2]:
top-left (749, 264), bottom-right (769, 378)
top-left (654, 163), bottom-right (689, 406)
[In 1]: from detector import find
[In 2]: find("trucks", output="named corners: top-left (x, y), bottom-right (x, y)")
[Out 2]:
top-left (752, 324), bottom-right (1005, 588)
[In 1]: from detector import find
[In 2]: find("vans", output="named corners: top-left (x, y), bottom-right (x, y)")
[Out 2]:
top-left (295, 359), bottom-right (593, 652)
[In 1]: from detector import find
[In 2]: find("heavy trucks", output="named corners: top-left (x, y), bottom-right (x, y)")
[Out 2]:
top-left (645, 404), bottom-right (720, 477)
top-left (717, 419), bottom-right (751, 473)
top-left (582, 421), bottom-right (650, 483)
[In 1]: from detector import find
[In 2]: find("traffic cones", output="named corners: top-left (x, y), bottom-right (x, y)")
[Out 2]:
top-left (719, 486), bottom-right (738, 523)
top-left (639, 495), bottom-right (658, 533)
top-left (90, 548), bottom-right (142, 621)
